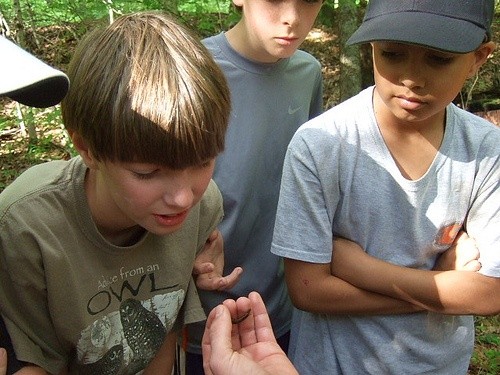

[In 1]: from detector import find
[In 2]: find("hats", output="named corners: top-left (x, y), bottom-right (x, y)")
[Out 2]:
top-left (347, 0), bottom-right (496, 54)
top-left (0, 34), bottom-right (71, 108)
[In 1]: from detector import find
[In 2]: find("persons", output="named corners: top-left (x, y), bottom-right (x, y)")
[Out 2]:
top-left (202, 291), bottom-right (298, 375)
top-left (184, 1), bottom-right (324, 375)
top-left (0, 37), bottom-right (70, 373)
top-left (0, 10), bottom-right (231, 375)
top-left (270, 0), bottom-right (500, 375)
